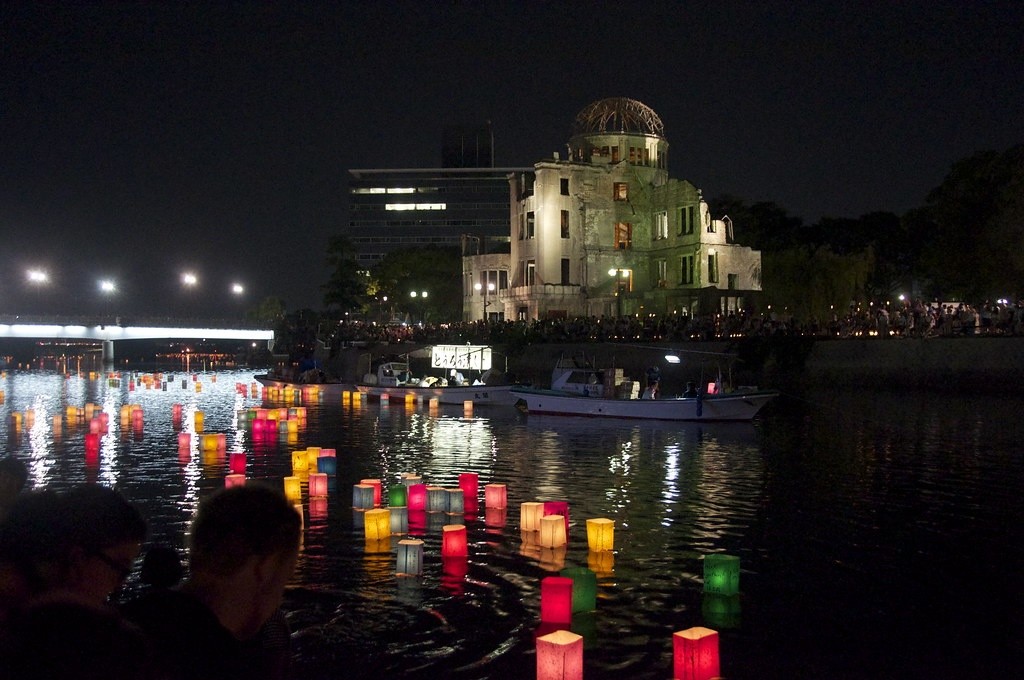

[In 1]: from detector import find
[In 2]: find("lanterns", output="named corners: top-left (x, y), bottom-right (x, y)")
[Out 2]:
top-left (703, 553), bottom-right (741, 597)
top-left (540, 578), bottom-right (574, 626)
top-left (559, 568), bottom-right (599, 614)
top-left (1, 349), bottom-right (475, 461)
top-left (535, 629), bottom-right (584, 680)
top-left (223, 446), bottom-right (615, 576)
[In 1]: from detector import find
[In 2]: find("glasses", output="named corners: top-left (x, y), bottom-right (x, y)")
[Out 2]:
top-left (86, 549), bottom-right (133, 582)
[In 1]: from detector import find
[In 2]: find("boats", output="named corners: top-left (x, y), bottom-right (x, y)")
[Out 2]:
top-left (510, 347), bottom-right (781, 422)
top-left (253, 362), bottom-right (350, 396)
top-left (353, 340), bottom-right (522, 407)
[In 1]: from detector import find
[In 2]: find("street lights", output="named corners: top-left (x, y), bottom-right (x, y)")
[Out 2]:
top-left (410, 290), bottom-right (428, 324)
top-left (474, 280), bottom-right (495, 323)
top-left (608, 265), bottom-right (630, 318)
top-left (374, 295), bottom-right (388, 323)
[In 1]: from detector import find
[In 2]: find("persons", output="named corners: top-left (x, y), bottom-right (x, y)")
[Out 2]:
top-left (0, 458), bottom-right (303, 680)
top-left (267, 297), bottom-right (1024, 349)
top-left (639, 363), bottom-right (698, 402)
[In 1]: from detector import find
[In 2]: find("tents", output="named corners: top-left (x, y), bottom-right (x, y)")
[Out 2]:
top-left (672, 626), bottom-right (722, 680)
